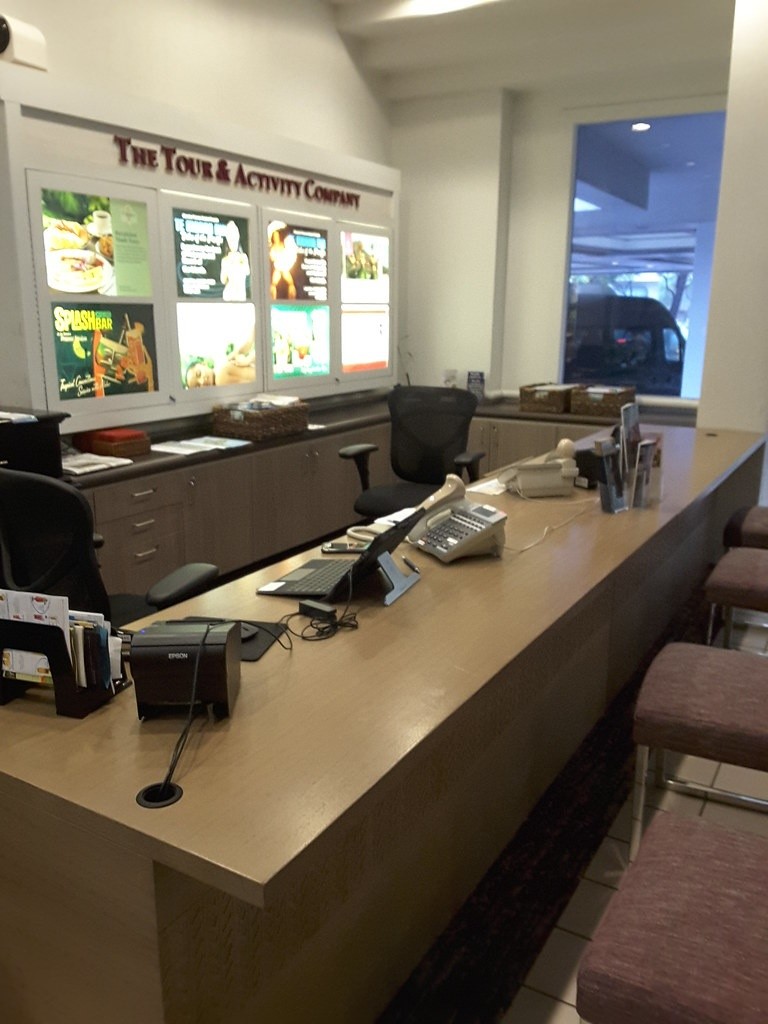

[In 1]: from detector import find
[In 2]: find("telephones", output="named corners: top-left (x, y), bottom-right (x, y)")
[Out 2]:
top-left (405, 473), bottom-right (508, 564)
top-left (495, 437), bottom-right (579, 499)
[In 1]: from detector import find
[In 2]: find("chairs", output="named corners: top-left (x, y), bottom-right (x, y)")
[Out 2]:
top-left (0, 470), bottom-right (220, 627)
top-left (339, 386), bottom-right (487, 520)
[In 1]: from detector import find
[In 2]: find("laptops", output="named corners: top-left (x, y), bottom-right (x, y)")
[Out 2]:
top-left (257, 508), bottom-right (426, 601)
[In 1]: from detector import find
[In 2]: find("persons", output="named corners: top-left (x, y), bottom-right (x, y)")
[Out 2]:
top-left (185, 357), bottom-right (255, 386)
top-left (221, 221), bottom-right (252, 301)
top-left (228, 328), bottom-right (256, 365)
top-left (359, 243), bottom-right (378, 279)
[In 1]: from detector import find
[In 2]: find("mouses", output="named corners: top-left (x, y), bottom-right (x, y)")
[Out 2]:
top-left (241, 623), bottom-right (259, 641)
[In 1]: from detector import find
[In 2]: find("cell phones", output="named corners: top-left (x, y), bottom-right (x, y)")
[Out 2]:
top-left (321, 542), bottom-right (367, 554)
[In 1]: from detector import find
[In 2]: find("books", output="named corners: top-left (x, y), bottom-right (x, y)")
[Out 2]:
top-left (3, 610), bottom-right (111, 689)
top-left (621, 402), bottom-right (664, 507)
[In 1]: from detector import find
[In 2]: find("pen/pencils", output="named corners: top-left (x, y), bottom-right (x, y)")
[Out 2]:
top-left (397, 548), bottom-right (421, 574)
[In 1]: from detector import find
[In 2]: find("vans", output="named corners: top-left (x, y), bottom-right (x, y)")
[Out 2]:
top-left (563, 295), bottom-right (684, 395)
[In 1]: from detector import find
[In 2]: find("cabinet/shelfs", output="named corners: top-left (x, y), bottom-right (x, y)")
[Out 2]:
top-left (81, 417), bottom-right (610, 597)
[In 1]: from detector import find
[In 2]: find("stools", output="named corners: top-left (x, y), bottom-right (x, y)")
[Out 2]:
top-left (576, 506), bottom-right (768, 1024)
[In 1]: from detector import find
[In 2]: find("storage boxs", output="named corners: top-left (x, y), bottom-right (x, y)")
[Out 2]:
top-left (86, 436), bottom-right (151, 459)
